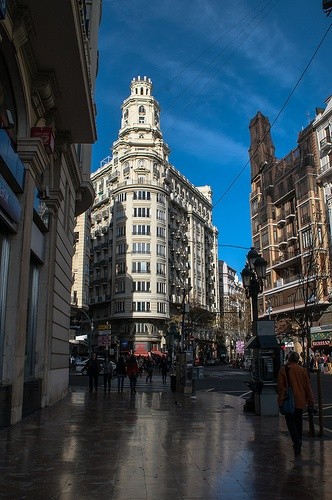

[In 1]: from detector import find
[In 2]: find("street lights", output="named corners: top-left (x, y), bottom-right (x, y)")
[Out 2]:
top-left (238, 246), bottom-right (268, 414)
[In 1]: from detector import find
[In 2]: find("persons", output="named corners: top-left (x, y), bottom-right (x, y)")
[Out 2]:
top-left (309, 348), bottom-right (332, 375)
top-left (127, 355), bottom-right (139, 393)
top-left (115, 356), bottom-right (126, 394)
top-left (286, 351), bottom-right (292, 364)
top-left (237, 362), bottom-right (245, 370)
top-left (99, 356), bottom-right (114, 395)
top-left (278, 352), bottom-right (315, 460)
top-left (135, 353), bottom-right (171, 384)
top-left (81, 352), bottom-right (100, 393)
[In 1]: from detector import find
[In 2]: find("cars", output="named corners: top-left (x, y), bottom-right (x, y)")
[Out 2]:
top-left (192, 356), bottom-right (221, 366)
top-left (75, 357), bottom-right (117, 376)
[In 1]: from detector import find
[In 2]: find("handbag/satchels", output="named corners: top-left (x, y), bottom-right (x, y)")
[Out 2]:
top-left (280, 389), bottom-right (295, 416)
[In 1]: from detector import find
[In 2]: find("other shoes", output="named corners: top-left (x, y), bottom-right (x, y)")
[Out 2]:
top-left (293, 439), bottom-right (302, 455)
